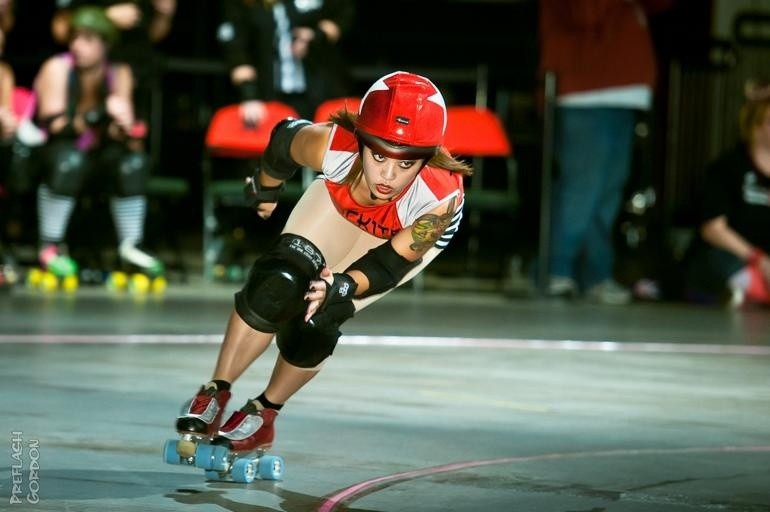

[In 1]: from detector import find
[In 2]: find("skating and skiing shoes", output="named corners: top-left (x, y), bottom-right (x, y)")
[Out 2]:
top-left (29, 238), bottom-right (78, 292)
top-left (163, 385), bottom-right (283, 483)
top-left (111, 246), bottom-right (167, 295)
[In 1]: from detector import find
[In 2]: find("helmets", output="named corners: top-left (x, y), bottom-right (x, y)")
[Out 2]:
top-left (353, 72), bottom-right (447, 160)
top-left (71, 5), bottom-right (121, 46)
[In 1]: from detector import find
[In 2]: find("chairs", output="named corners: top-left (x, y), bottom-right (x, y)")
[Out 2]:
top-left (5, 84), bottom-right (522, 294)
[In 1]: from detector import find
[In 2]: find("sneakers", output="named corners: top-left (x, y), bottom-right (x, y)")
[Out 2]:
top-left (544, 274), bottom-right (633, 306)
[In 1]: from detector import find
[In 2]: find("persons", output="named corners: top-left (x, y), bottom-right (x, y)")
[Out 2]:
top-left (171, 68), bottom-right (473, 459)
top-left (2, 0), bottom-right (770, 313)
